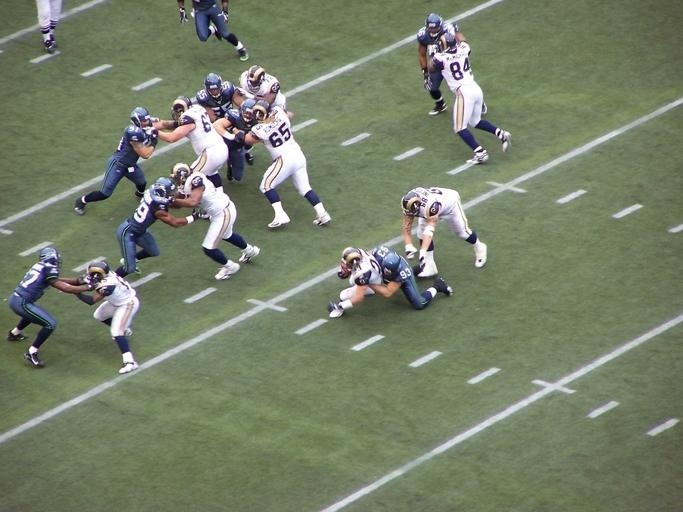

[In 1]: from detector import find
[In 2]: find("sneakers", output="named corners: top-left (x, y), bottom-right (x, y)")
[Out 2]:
top-left (428, 103), bottom-right (448, 116)
top-left (210, 23), bottom-right (223, 41)
top-left (236, 46), bottom-right (248, 61)
top-left (436, 276), bottom-right (453, 297)
top-left (481, 102), bottom-right (487, 115)
top-left (43, 39), bottom-right (56, 54)
top-left (473, 242), bottom-right (487, 268)
top-left (418, 264), bottom-right (438, 278)
top-left (467, 150), bottom-right (490, 165)
top-left (499, 130), bottom-right (511, 153)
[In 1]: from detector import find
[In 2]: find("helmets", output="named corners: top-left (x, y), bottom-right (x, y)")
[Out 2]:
top-left (170, 161), bottom-right (193, 185)
top-left (130, 104), bottom-right (152, 129)
top-left (39, 247), bottom-right (63, 271)
top-left (341, 246), bottom-right (362, 272)
top-left (169, 64), bottom-right (270, 122)
top-left (401, 192), bottom-right (426, 218)
top-left (85, 259), bottom-right (110, 284)
top-left (437, 33), bottom-right (457, 52)
top-left (154, 177), bottom-right (179, 200)
top-left (382, 253), bottom-right (401, 279)
top-left (424, 12), bottom-right (443, 38)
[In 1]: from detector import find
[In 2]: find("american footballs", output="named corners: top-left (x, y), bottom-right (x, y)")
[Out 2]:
top-left (172, 191), bottom-right (185, 209)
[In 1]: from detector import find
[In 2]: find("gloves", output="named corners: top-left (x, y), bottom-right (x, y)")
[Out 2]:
top-left (192, 207), bottom-right (210, 221)
top-left (89, 280), bottom-right (102, 293)
top-left (423, 77), bottom-right (432, 93)
top-left (179, 7), bottom-right (189, 25)
top-left (234, 131), bottom-right (245, 144)
top-left (150, 128), bottom-right (158, 137)
top-left (217, 7), bottom-right (230, 24)
top-left (419, 250), bottom-right (426, 264)
top-left (405, 246), bottom-right (417, 260)
top-left (328, 303), bottom-right (343, 312)
top-left (78, 273), bottom-right (91, 284)
top-left (452, 24), bottom-right (459, 33)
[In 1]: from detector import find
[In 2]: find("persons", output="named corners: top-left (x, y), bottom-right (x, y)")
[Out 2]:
top-left (398, 185), bottom-right (487, 280)
top-left (9, 249), bottom-right (96, 371)
top-left (74, 261), bottom-right (140, 376)
top-left (31, 0), bottom-right (69, 52)
top-left (328, 246), bottom-right (454, 319)
top-left (178, 0), bottom-right (249, 61)
top-left (417, 13), bottom-right (511, 166)
top-left (76, 66), bottom-right (331, 282)
